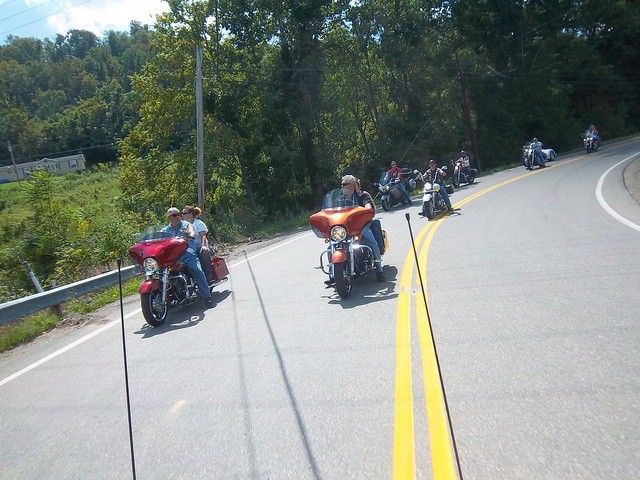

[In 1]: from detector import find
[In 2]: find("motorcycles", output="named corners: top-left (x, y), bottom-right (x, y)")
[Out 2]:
top-left (523, 146), bottom-right (555, 166)
top-left (375, 168), bottom-right (415, 211)
top-left (309, 189), bottom-right (389, 298)
top-left (129, 224), bottom-right (228, 327)
top-left (413, 166), bottom-right (454, 219)
top-left (582, 131), bottom-right (599, 152)
top-left (450, 159), bottom-right (478, 188)
top-left (525, 144), bottom-right (547, 170)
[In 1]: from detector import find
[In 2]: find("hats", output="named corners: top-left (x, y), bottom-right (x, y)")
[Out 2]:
top-left (429, 160), bottom-right (436, 163)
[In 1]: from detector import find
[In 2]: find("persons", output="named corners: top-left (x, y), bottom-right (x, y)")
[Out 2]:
top-left (323, 175), bottom-right (387, 285)
top-left (158, 207), bottom-right (212, 306)
top-left (384, 161), bottom-right (413, 206)
top-left (354, 175), bottom-right (386, 255)
top-left (456, 150), bottom-right (472, 182)
top-left (530, 137), bottom-right (545, 167)
top-left (588, 124), bottom-right (602, 147)
top-left (417, 160), bottom-right (453, 216)
top-left (181, 206), bottom-right (215, 286)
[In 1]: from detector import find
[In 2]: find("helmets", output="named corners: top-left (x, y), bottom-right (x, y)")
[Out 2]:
top-left (461, 150), bottom-right (467, 154)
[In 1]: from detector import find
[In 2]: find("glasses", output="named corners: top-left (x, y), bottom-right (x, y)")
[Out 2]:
top-left (170, 212), bottom-right (181, 218)
top-left (392, 164), bottom-right (396, 165)
top-left (341, 182), bottom-right (352, 186)
top-left (182, 212), bottom-right (189, 215)
top-left (429, 163), bottom-right (435, 166)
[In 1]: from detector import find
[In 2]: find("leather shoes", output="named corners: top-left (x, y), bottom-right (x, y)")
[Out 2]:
top-left (377, 272), bottom-right (387, 282)
top-left (204, 297), bottom-right (215, 308)
top-left (324, 278), bottom-right (334, 285)
